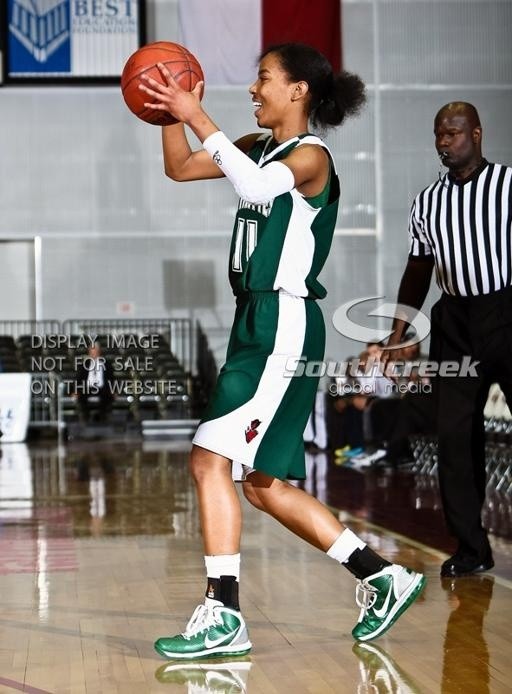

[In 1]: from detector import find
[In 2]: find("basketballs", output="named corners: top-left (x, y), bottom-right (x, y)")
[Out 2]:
top-left (121, 40), bottom-right (204, 126)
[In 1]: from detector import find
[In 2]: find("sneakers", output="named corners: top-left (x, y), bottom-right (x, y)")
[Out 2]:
top-left (155, 596), bottom-right (252, 661)
top-left (441, 550), bottom-right (494, 577)
top-left (351, 564), bottom-right (427, 642)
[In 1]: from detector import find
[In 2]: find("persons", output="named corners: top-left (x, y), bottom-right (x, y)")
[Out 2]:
top-left (74, 451), bottom-right (118, 539)
top-left (71, 342), bottom-right (116, 426)
top-left (155, 642), bottom-right (424, 694)
top-left (380, 102), bottom-right (512, 575)
top-left (137, 43), bottom-right (427, 661)
top-left (439, 574), bottom-right (495, 694)
top-left (326, 328), bottom-right (432, 467)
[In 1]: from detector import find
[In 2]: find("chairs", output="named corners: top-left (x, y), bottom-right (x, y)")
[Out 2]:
top-left (406, 381), bottom-right (511, 494)
top-left (0, 332), bottom-right (198, 435)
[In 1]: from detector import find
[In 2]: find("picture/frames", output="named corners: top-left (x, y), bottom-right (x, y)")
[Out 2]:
top-left (0, 0), bottom-right (148, 88)
